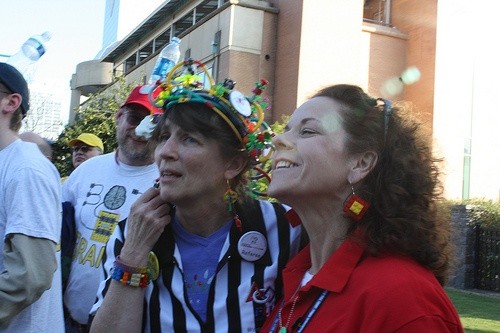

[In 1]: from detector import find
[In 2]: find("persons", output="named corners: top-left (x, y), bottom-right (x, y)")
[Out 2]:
top-left (61, 133), bottom-right (104, 184)
top-left (18, 131), bottom-right (53, 160)
top-left (0, 62), bottom-right (67, 333)
top-left (60, 82), bottom-right (162, 333)
top-left (85, 80), bottom-right (308, 333)
top-left (259, 85), bottom-right (466, 333)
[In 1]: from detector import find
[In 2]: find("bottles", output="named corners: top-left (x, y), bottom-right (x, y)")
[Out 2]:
top-left (148, 36), bottom-right (182, 87)
top-left (5, 30), bottom-right (52, 75)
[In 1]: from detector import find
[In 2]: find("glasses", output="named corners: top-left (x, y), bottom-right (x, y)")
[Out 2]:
top-left (121, 110), bottom-right (144, 125)
top-left (71, 145), bottom-right (98, 153)
top-left (374, 97), bottom-right (395, 165)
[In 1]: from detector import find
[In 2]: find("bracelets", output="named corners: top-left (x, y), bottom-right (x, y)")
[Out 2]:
top-left (114, 255), bottom-right (149, 274)
top-left (111, 261), bottom-right (150, 289)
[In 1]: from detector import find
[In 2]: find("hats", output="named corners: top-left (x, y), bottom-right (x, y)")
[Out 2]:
top-left (68, 133), bottom-right (104, 153)
top-left (0, 62), bottom-right (30, 119)
top-left (120, 86), bottom-right (164, 114)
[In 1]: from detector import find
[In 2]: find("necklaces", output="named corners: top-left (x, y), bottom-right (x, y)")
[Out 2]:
top-left (278, 295), bottom-right (299, 333)
